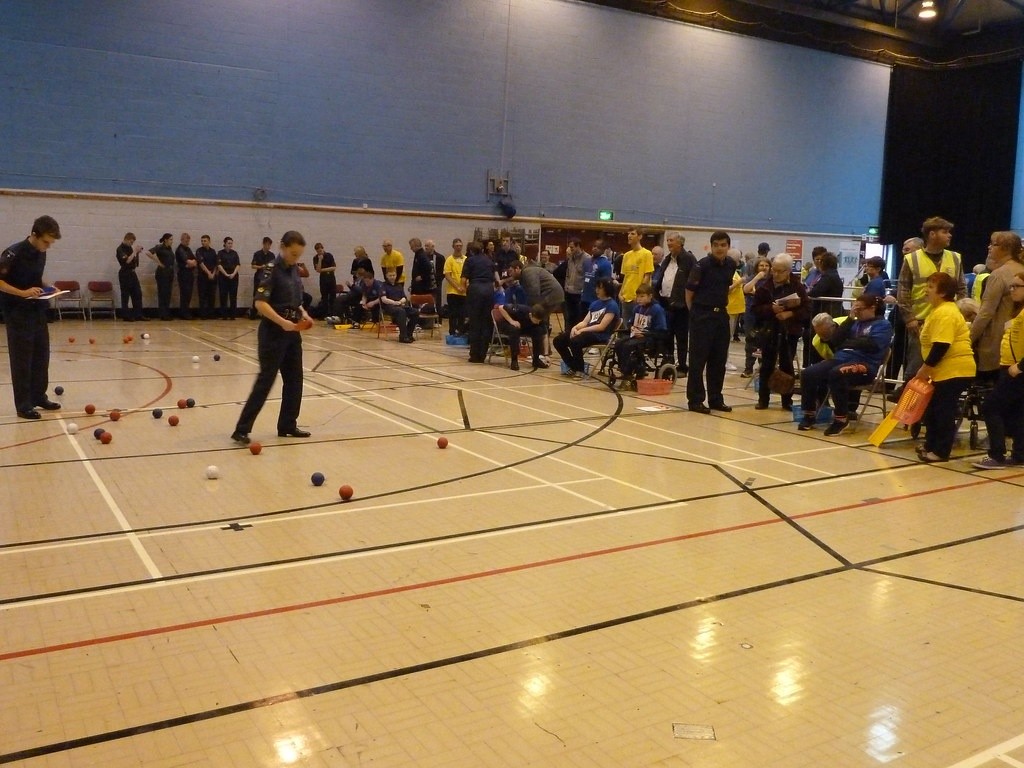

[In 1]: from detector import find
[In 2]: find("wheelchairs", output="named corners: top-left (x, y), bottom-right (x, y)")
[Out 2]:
top-left (909, 376), bottom-right (985, 450)
top-left (607, 301), bottom-right (677, 392)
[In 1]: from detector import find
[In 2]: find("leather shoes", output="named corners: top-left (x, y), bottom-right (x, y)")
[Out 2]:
top-left (511, 360), bottom-right (520, 371)
top-left (17, 399), bottom-right (61, 420)
top-left (400, 336), bottom-right (416, 343)
top-left (689, 403), bottom-right (732, 414)
top-left (230, 430), bottom-right (250, 444)
top-left (532, 358), bottom-right (548, 369)
top-left (278, 428), bottom-right (311, 438)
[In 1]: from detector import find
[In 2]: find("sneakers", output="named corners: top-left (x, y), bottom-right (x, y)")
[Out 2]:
top-left (559, 369), bottom-right (585, 381)
top-left (798, 416), bottom-right (814, 430)
top-left (824, 419), bottom-right (851, 436)
top-left (611, 379), bottom-right (631, 391)
top-left (971, 457), bottom-right (1024, 469)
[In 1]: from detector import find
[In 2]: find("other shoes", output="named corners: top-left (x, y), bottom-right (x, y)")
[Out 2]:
top-left (725, 362), bottom-right (738, 371)
top-left (783, 403), bottom-right (794, 411)
top-left (123, 314), bottom-right (152, 321)
top-left (741, 370), bottom-right (752, 379)
top-left (755, 404), bottom-right (769, 410)
top-left (670, 370), bottom-right (687, 379)
top-left (915, 444), bottom-right (949, 462)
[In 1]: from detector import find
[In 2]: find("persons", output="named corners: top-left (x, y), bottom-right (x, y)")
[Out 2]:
top-left (315, 215), bottom-right (1024, 474)
top-left (460, 242), bottom-right (495, 364)
top-left (117, 232), bottom-right (150, 322)
top-left (297, 263), bottom-right (313, 314)
top-left (143, 232), bottom-right (242, 320)
top-left (230, 230), bottom-right (314, 443)
top-left (379, 267), bottom-right (421, 344)
top-left (551, 277), bottom-right (621, 381)
top-left (913, 272), bottom-right (975, 463)
top-left (0, 215), bottom-right (70, 421)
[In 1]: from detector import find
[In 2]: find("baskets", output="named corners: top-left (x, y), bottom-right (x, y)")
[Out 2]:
top-left (560, 359), bottom-right (589, 375)
top-left (792, 405), bottom-right (832, 424)
top-left (335, 322), bottom-right (375, 329)
top-left (445, 335), bottom-right (468, 346)
top-left (637, 378), bottom-right (674, 394)
top-left (891, 377), bottom-right (934, 425)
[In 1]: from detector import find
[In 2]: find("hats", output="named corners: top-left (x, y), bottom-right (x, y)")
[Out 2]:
top-left (758, 242), bottom-right (771, 250)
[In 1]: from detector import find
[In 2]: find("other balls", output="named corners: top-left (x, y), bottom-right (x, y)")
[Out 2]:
top-left (69, 337), bottom-right (75, 342)
top-left (168, 415), bottom-right (179, 426)
top-left (437, 437), bottom-right (448, 448)
top-left (339, 485), bottom-right (353, 499)
top-left (55, 386), bottom-right (64, 395)
top-left (214, 354), bottom-right (220, 361)
top-left (110, 411), bottom-right (120, 421)
top-left (206, 465), bottom-right (218, 478)
top-left (250, 443), bottom-right (261, 455)
top-left (178, 398), bottom-right (195, 409)
top-left (94, 428), bottom-right (112, 444)
top-left (85, 404), bottom-right (95, 414)
top-left (67, 423), bottom-right (78, 433)
top-left (152, 408), bottom-right (163, 418)
top-left (144, 334), bottom-right (149, 338)
top-left (192, 355), bottom-right (200, 363)
top-left (311, 472), bottom-right (325, 486)
top-left (89, 338), bottom-right (95, 343)
top-left (123, 335), bottom-right (133, 343)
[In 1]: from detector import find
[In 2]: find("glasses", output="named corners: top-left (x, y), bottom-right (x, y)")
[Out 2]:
top-left (989, 242), bottom-right (1001, 247)
top-left (854, 307), bottom-right (869, 312)
top-left (1009, 283), bottom-right (1024, 289)
top-left (771, 267), bottom-right (787, 274)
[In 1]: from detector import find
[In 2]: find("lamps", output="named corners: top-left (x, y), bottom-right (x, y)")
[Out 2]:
top-left (919, 0), bottom-right (936, 18)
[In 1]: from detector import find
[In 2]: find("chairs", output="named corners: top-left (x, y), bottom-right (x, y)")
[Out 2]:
top-left (54, 281), bottom-right (87, 322)
top-left (88, 282), bottom-right (117, 320)
top-left (333, 283), bottom-right (905, 436)
